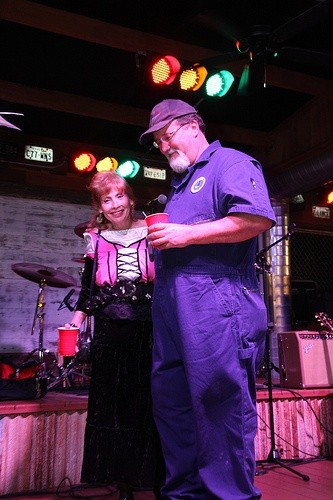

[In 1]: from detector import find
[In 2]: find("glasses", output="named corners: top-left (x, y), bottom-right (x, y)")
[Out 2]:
top-left (153, 123), bottom-right (188, 148)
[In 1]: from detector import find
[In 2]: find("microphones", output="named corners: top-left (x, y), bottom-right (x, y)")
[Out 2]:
top-left (59, 289), bottom-right (75, 309)
top-left (146, 194), bottom-right (168, 207)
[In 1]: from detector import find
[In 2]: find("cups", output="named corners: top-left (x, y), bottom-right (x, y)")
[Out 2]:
top-left (145, 213), bottom-right (169, 249)
top-left (57, 326), bottom-right (80, 357)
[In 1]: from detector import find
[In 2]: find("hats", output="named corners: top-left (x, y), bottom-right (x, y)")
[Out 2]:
top-left (138, 99), bottom-right (198, 144)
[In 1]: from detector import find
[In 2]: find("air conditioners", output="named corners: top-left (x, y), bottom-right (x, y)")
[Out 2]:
top-left (173, 61), bottom-right (209, 96)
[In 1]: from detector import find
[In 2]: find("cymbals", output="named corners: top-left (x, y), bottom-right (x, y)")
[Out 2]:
top-left (74, 221), bottom-right (91, 239)
top-left (11, 262), bottom-right (76, 289)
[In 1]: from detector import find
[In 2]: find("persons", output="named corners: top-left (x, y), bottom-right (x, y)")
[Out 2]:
top-left (140, 99), bottom-right (277, 500)
top-left (65, 171), bottom-right (165, 500)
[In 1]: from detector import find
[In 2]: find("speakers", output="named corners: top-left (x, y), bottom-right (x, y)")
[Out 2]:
top-left (277, 331), bottom-right (333, 389)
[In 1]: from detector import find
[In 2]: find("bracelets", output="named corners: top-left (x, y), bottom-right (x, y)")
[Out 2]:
top-left (70, 323), bottom-right (77, 328)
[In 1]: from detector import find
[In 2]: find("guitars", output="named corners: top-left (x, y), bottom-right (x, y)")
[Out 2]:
top-left (314, 310), bottom-right (333, 332)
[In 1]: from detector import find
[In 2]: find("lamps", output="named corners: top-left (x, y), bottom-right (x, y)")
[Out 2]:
top-left (143, 53), bottom-right (182, 86)
top-left (200, 68), bottom-right (236, 101)
top-left (73, 151), bottom-right (144, 181)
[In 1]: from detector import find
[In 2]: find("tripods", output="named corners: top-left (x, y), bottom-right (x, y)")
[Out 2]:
top-left (255, 222), bottom-right (310, 482)
top-left (0, 277), bottom-right (72, 399)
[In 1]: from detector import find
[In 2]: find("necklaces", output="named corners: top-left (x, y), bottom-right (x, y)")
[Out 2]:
top-left (111, 224), bottom-right (132, 235)
